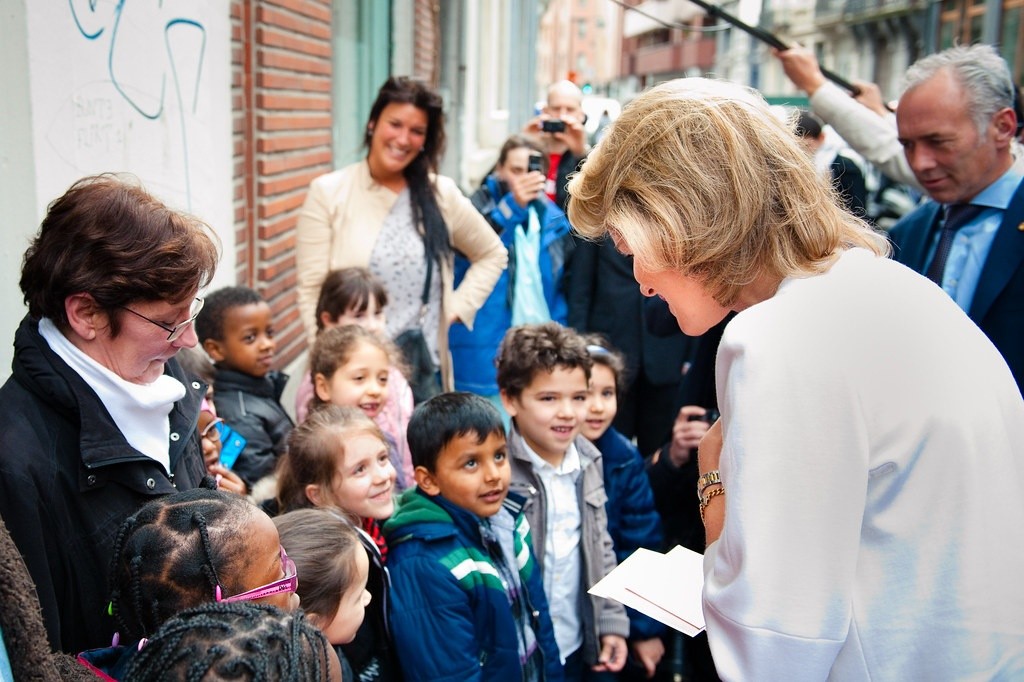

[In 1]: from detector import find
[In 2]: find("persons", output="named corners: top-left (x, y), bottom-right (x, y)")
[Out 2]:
top-left (0, 77), bottom-right (738, 682)
top-left (885, 42), bottom-right (1024, 398)
top-left (566, 78), bottom-right (1024, 682)
top-left (793, 115), bottom-right (865, 218)
top-left (774, 45), bottom-right (1024, 198)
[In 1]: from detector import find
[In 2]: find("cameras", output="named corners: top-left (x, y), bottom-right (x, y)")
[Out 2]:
top-left (691, 409), bottom-right (721, 427)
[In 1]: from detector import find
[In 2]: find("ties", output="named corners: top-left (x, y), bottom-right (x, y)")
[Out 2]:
top-left (925, 204), bottom-right (988, 287)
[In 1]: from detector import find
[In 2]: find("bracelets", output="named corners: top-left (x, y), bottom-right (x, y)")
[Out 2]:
top-left (697, 470), bottom-right (720, 501)
top-left (700, 487), bottom-right (724, 522)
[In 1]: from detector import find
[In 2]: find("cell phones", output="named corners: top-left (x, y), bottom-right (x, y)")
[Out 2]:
top-left (542, 121), bottom-right (564, 133)
top-left (527, 155), bottom-right (542, 173)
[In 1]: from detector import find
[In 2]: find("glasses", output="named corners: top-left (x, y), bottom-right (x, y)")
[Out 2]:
top-left (220, 544), bottom-right (298, 603)
top-left (114, 295), bottom-right (206, 341)
